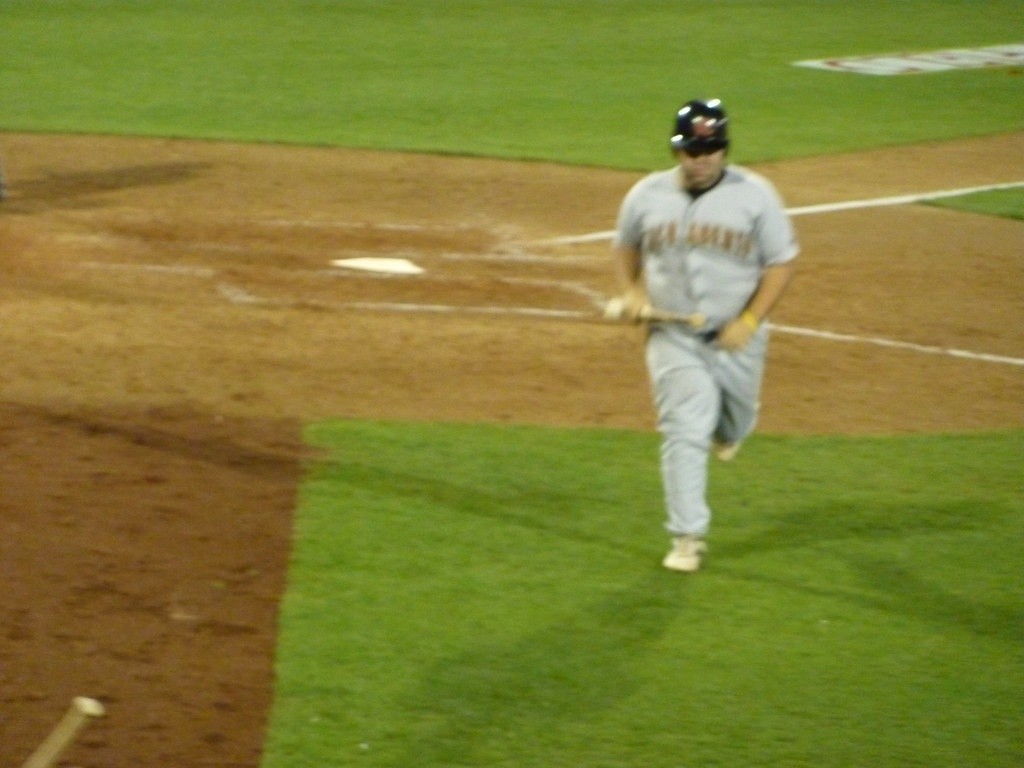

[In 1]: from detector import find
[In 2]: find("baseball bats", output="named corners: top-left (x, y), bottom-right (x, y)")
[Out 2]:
top-left (599, 298), bottom-right (707, 332)
top-left (20, 694), bottom-right (110, 768)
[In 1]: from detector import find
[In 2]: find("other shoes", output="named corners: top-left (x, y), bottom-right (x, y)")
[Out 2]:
top-left (663, 536), bottom-right (706, 572)
top-left (713, 440), bottom-right (738, 460)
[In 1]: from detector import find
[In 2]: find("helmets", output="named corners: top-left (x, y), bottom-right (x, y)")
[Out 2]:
top-left (670, 101), bottom-right (729, 149)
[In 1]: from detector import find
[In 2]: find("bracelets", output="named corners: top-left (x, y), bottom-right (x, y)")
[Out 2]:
top-left (740, 313), bottom-right (760, 330)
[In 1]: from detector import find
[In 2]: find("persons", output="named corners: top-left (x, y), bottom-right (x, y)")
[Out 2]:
top-left (613, 100), bottom-right (799, 572)
